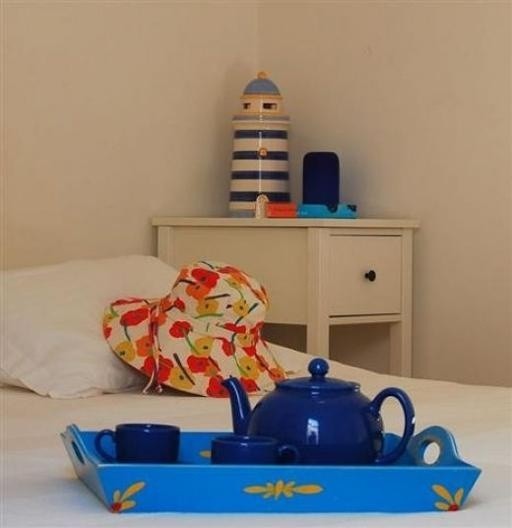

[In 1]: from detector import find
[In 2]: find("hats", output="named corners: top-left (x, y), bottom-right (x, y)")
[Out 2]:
top-left (101, 262), bottom-right (287, 396)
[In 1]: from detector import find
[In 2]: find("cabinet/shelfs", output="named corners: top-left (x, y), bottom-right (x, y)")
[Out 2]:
top-left (153, 215), bottom-right (419, 377)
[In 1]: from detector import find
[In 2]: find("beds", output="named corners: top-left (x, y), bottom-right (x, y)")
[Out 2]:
top-left (0, 341), bottom-right (512, 526)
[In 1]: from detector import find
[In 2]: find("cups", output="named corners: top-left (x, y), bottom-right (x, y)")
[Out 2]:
top-left (96, 422), bottom-right (179, 464)
top-left (210, 435), bottom-right (300, 466)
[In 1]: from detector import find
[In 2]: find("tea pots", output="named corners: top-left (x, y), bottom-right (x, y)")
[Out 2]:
top-left (220, 357), bottom-right (416, 466)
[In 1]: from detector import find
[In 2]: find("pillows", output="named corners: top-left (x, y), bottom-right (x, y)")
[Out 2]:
top-left (0, 254), bottom-right (181, 398)
